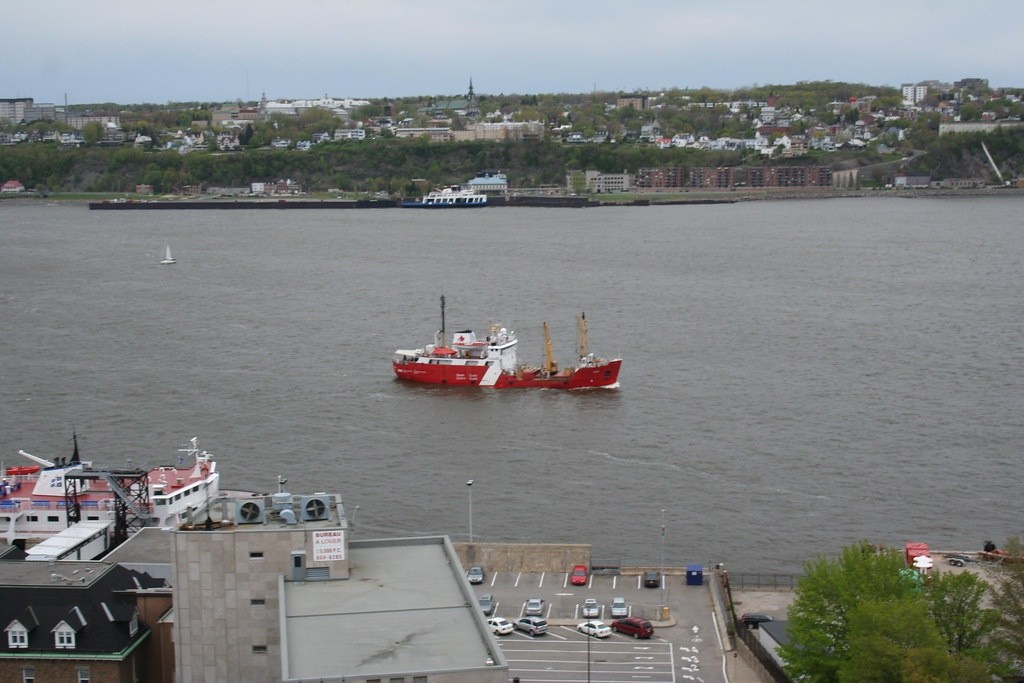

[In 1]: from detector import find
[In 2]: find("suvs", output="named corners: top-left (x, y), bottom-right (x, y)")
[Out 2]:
top-left (611, 616), bottom-right (654, 640)
top-left (610, 598), bottom-right (629, 618)
top-left (581, 599), bottom-right (601, 618)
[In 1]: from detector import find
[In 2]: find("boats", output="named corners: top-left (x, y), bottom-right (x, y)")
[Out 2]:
top-left (391, 292), bottom-right (624, 391)
top-left (396, 182), bottom-right (488, 208)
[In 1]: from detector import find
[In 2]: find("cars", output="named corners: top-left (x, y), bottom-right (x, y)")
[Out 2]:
top-left (487, 617), bottom-right (515, 636)
top-left (744, 615), bottom-right (772, 631)
top-left (577, 621), bottom-right (613, 638)
top-left (479, 594), bottom-right (496, 615)
top-left (643, 569), bottom-right (661, 588)
top-left (466, 566), bottom-right (485, 584)
top-left (526, 598), bottom-right (545, 616)
top-left (570, 565), bottom-right (588, 586)
top-left (513, 615), bottom-right (549, 637)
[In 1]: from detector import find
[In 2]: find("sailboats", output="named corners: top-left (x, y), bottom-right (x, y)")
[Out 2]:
top-left (159, 243), bottom-right (177, 265)
